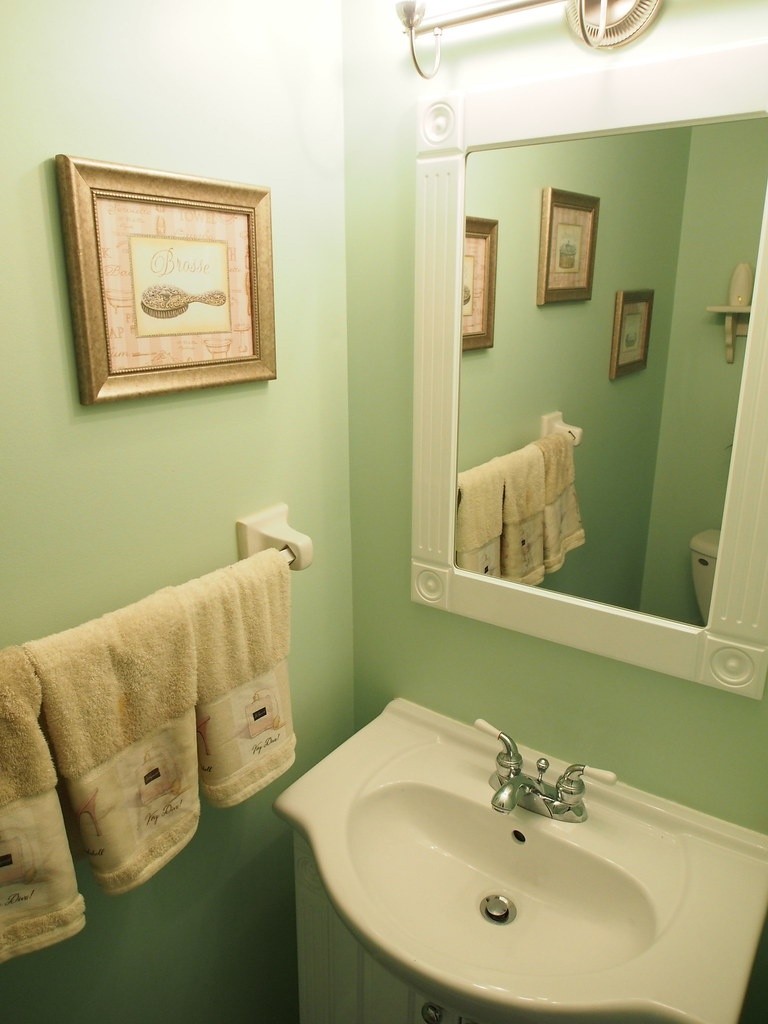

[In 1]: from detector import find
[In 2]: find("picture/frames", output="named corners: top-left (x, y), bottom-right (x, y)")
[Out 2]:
top-left (56, 154), bottom-right (277, 404)
top-left (534, 185), bottom-right (601, 304)
top-left (459, 216), bottom-right (498, 350)
top-left (609, 288), bottom-right (654, 375)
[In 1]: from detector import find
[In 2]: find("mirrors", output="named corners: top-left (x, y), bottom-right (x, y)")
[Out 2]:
top-left (412, 35), bottom-right (768, 700)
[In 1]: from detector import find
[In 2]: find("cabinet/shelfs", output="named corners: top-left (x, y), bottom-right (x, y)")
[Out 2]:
top-left (294, 829), bottom-right (472, 1024)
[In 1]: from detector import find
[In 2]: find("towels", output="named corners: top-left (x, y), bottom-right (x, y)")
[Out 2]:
top-left (0, 643), bottom-right (88, 963)
top-left (170, 548), bottom-right (297, 810)
top-left (492, 443), bottom-right (547, 586)
top-left (530, 430), bottom-right (586, 575)
top-left (18, 585), bottom-right (203, 899)
top-left (455, 456), bottom-right (505, 578)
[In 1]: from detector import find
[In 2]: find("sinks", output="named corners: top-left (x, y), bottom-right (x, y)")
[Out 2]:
top-left (273, 697), bottom-right (768, 1024)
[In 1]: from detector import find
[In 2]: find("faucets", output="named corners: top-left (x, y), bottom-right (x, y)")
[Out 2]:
top-left (473, 718), bottom-right (619, 824)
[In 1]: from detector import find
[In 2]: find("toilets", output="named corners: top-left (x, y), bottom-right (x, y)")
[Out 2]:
top-left (688, 529), bottom-right (721, 628)
